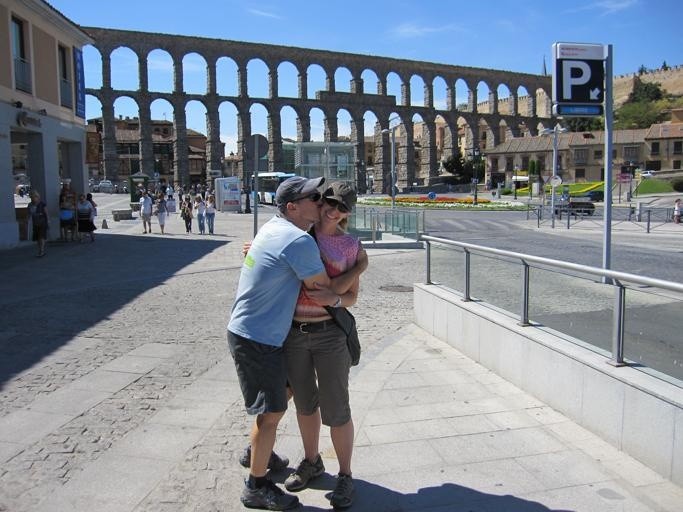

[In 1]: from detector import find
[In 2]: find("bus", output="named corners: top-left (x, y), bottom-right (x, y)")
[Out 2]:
top-left (249, 172), bottom-right (296, 206)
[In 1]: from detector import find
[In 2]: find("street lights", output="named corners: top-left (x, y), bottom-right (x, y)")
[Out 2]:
top-left (538, 126), bottom-right (568, 215)
top-left (380, 127), bottom-right (395, 210)
top-left (513, 163), bottom-right (519, 200)
top-left (465, 144), bottom-right (487, 204)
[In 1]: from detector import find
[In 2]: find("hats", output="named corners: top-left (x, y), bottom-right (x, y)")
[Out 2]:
top-left (274, 176), bottom-right (325, 207)
top-left (323, 180), bottom-right (355, 211)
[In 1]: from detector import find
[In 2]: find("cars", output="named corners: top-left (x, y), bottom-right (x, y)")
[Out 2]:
top-left (553, 195), bottom-right (595, 216)
top-left (640, 170), bottom-right (654, 180)
top-left (584, 191), bottom-right (604, 201)
top-left (88, 178), bottom-right (128, 194)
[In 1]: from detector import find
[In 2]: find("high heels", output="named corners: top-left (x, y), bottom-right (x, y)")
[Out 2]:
top-left (37, 248), bottom-right (48, 257)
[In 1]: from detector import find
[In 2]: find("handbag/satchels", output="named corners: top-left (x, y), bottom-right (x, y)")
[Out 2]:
top-left (323, 305), bottom-right (361, 366)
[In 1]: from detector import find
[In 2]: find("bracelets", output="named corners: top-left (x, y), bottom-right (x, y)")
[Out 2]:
top-left (333, 296), bottom-right (342, 308)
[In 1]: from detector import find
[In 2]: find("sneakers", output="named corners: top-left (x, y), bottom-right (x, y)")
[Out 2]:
top-left (240, 479), bottom-right (299, 511)
top-left (283, 456), bottom-right (325, 492)
top-left (78, 234), bottom-right (96, 244)
top-left (239, 446), bottom-right (289, 470)
top-left (330, 474), bottom-right (355, 508)
top-left (184, 228), bottom-right (213, 235)
top-left (142, 230), bottom-right (164, 235)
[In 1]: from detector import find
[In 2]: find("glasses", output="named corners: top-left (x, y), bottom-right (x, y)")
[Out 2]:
top-left (322, 196), bottom-right (347, 214)
top-left (291, 192), bottom-right (321, 204)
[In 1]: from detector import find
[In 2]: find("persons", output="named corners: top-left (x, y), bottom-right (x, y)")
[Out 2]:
top-left (242, 181), bottom-right (361, 509)
top-left (674, 199), bottom-right (682, 224)
top-left (112, 183), bottom-right (128, 194)
top-left (227, 176), bottom-right (369, 511)
top-left (137, 182), bottom-right (216, 235)
top-left (14, 177), bottom-right (98, 257)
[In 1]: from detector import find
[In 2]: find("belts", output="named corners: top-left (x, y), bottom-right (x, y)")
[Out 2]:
top-left (292, 318), bottom-right (334, 333)
